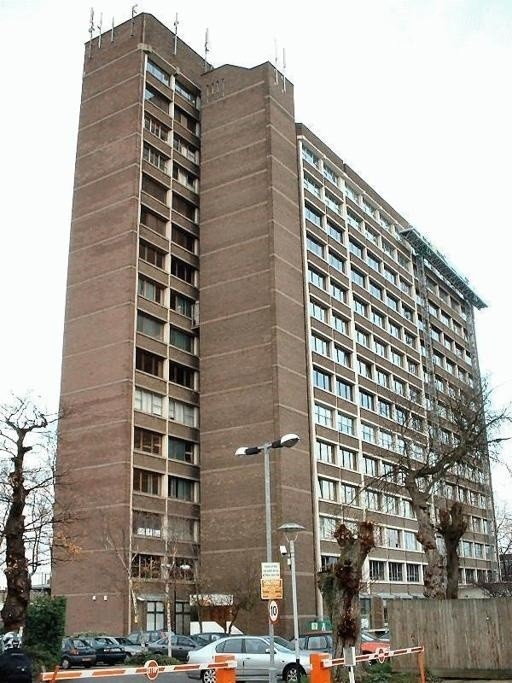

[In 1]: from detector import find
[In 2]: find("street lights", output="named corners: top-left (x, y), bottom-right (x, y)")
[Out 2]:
top-left (233, 432), bottom-right (301, 682)
top-left (160, 563), bottom-right (191, 634)
top-left (274, 521), bottom-right (307, 682)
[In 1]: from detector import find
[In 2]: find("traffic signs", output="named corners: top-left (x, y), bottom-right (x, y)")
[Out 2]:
top-left (269, 598), bottom-right (280, 624)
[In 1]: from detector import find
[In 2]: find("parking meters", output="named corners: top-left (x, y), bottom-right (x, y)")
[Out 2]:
top-left (342, 645), bottom-right (357, 682)
